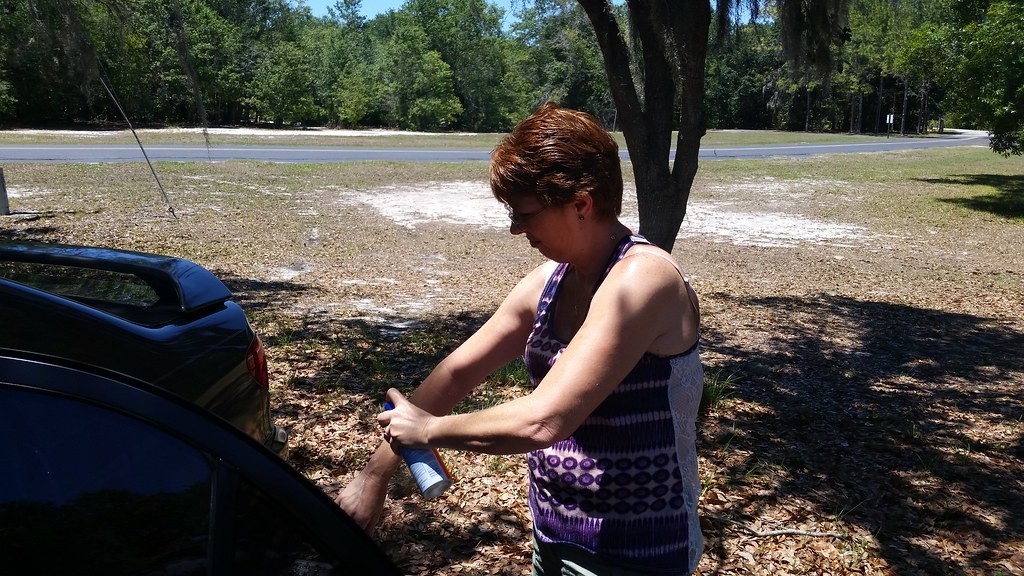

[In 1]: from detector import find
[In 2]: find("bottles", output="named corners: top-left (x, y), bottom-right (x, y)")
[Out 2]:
top-left (384, 403), bottom-right (453, 498)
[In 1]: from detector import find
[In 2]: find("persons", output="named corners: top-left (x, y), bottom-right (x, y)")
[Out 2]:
top-left (333, 102), bottom-right (704, 576)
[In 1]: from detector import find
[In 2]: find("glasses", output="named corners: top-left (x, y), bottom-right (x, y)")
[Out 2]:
top-left (503, 201), bottom-right (553, 232)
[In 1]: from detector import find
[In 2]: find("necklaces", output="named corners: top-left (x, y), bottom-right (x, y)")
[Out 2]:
top-left (572, 274), bottom-right (601, 319)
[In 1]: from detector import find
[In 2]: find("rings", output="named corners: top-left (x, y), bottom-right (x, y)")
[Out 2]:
top-left (384, 433), bottom-right (391, 439)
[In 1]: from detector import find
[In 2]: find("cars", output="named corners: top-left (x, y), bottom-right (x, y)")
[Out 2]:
top-left (0, 234), bottom-right (413, 576)
